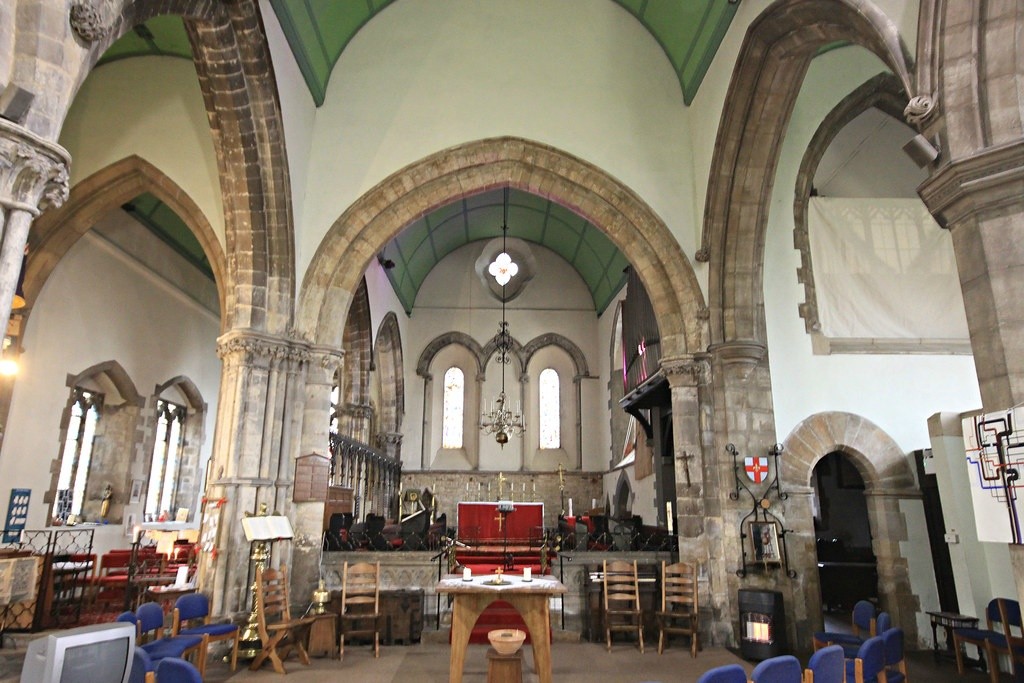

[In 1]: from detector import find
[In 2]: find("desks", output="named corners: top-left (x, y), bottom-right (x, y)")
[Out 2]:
top-left (926, 610), bottom-right (986, 674)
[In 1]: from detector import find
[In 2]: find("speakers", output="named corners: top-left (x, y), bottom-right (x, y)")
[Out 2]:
top-left (739, 587), bottom-right (785, 661)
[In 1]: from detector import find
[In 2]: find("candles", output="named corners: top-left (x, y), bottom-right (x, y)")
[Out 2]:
top-left (488, 481), bottom-right (490, 491)
top-left (510, 482), bottom-right (514, 490)
top-left (466, 482), bottom-right (469, 491)
top-left (522, 482), bottom-right (526, 491)
top-left (533, 482), bottom-right (536, 491)
top-left (477, 482), bottom-right (480, 491)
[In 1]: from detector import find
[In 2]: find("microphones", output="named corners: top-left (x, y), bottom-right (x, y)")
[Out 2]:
top-left (398, 491), bottom-right (407, 517)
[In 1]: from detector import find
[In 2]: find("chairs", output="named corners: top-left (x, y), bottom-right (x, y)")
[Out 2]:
top-left (156, 656), bottom-right (203, 683)
top-left (126, 646), bottom-right (155, 683)
top-left (655, 560), bottom-right (703, 659)
top-left (48, 538), bottom-right (199, 624)
top-left (248, 564), bottom-right (318, 674)
top-left (171, 593), bottom-right (240, 682)
top-left (984, 598), bottom-right (1024, 683)
top-left (952, 597), bottom-right (1024, 677)
top-left (602, 559), bottom-right (644, 654)
top-left (694, 601), bottom-right (908, 683)
top-left (116, 610), bottom-right (165, 683)
top-left (339, 559), bottom-right (383, 660)
top-left (135, 602), bottom-right (206, 675)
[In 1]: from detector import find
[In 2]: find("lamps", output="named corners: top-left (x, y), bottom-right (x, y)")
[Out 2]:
top-left (476, 284), bottom-right (527, 451)
top-left (487, 187), bottom-right (519, 285)
